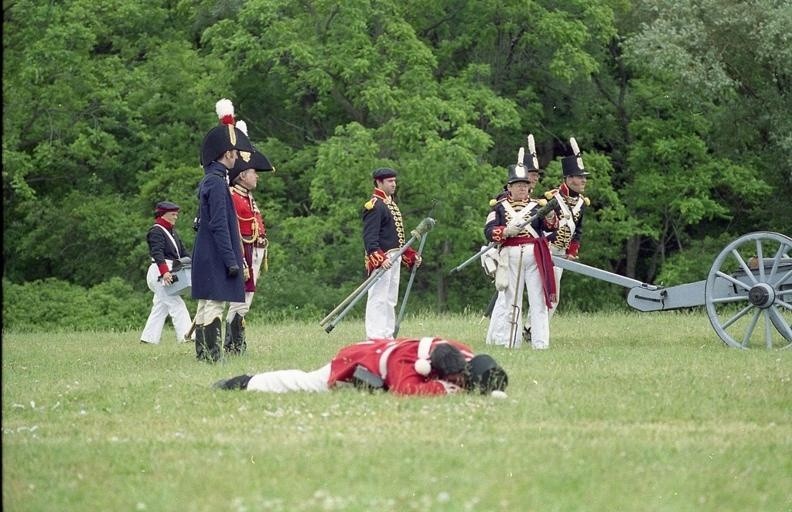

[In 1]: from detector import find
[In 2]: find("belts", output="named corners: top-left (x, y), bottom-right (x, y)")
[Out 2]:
top-left (243, 236), bottom-right (266, 247)
top-left (378, 339), bottom-right (420, 381)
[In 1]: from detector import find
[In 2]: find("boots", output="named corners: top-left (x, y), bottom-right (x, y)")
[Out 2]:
top-left (213, 374), bottom-right (253, 390)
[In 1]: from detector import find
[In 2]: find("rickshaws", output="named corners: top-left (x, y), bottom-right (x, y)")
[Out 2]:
top-left (548, 228), bottom-right (792, 354)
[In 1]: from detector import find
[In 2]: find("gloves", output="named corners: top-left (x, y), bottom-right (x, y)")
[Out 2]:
top-left (505, 223), bottom-right (525, 237)
top-left (243, 268), bottom-right (250, 282)
top-left (229, 265), bottom-right (240, 276)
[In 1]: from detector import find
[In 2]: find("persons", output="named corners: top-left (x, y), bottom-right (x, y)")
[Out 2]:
top-left (222, 119), bottom-right (276, 356)
top-left (190, 97), bottom-right (255, 364)
top-left (138, 201), bottom-right (196, 344)
top-left (361, 168), bottom-right (423, 340)
top-left (213, 337), bottom-right (510, 397)
top-left (484, 133), bottom-right (592, 351)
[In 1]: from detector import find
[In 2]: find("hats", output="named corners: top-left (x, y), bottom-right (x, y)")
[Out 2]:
top-left (507, 154), bottom-right (590, 185)
top-left (374, 168), bottom-right (396, 178)
top-left (155, 202), bottom-right (180, 215)
top-left (199, 126), bottom-right (254, 167)
top-left (462, 354), bottom-right (508, 393)
top-left (229, 148), bottom-right (274, 183)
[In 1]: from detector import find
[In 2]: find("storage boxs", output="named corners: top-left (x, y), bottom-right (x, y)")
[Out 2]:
top-left (158, 262), bottom-right (195, 295)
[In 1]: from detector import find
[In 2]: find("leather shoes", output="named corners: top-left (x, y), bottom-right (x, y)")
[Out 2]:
top-left (523, 332), bottom-right (531, 342)
